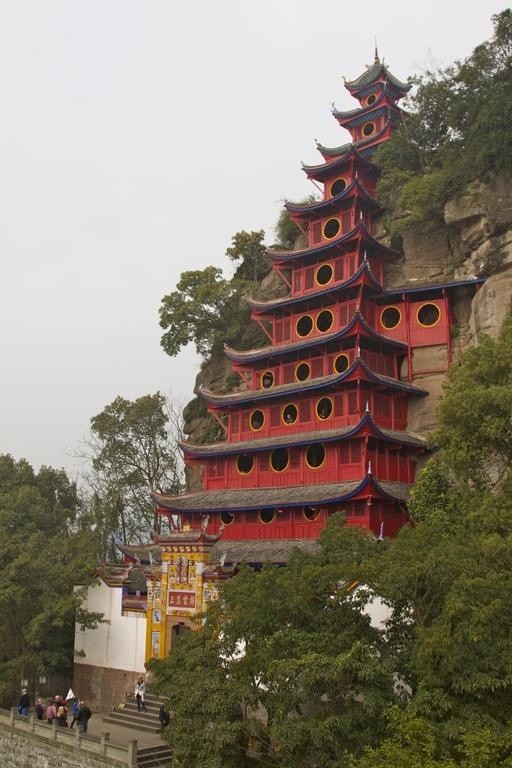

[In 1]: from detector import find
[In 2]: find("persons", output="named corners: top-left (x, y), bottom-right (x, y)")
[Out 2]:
top-left (133, 675), bottom-right (148, 715)
top-left (159, 703), bottom-right (170, 734)
top-left (16, 687), bottom-right (93, 733)
